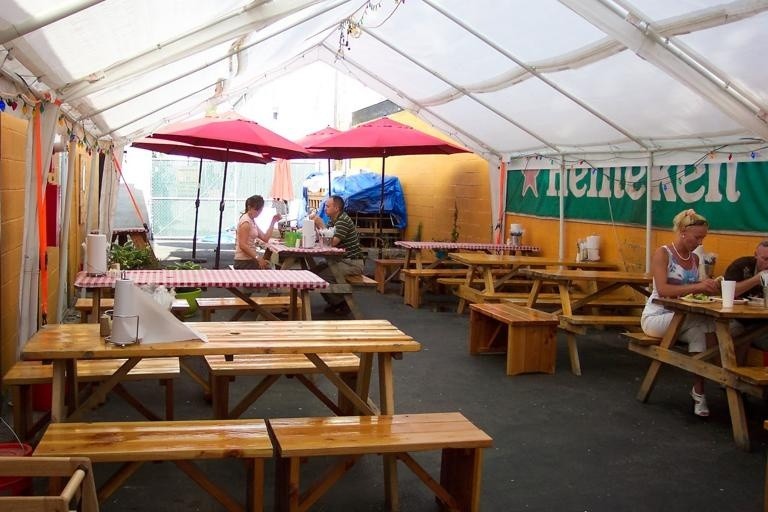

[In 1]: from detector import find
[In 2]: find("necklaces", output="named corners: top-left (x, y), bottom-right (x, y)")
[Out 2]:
top-left (670, 241), bottom-right (691, 262)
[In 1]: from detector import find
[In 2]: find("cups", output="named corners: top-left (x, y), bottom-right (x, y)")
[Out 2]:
top-left (110, 262), bottom-right (121, 279)
top-left (763, 285), bottom-right (768, 307)
top-left (720, 280), bottom-right (735, 307)
top-left (322, 236), bottom-right (333, 249)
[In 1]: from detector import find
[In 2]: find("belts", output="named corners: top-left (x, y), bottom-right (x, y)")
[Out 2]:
top-left (349, 255), bottom-right (364, 260)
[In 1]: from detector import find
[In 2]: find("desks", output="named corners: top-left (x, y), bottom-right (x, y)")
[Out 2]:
top-left (518, 268), bottom-right (654, 376)
top-left (74, 268), bottom-right (329, 384)
top-left (255, 236), bottom-right (364, 320)
top-left (18, 319), bottom-right (422, 511)
top-left (636, 294), bottom-right (767, 451)
top-left (447, 251), bottom-right (617, 317)
top-left (395, 242), bottom-right (539, 305)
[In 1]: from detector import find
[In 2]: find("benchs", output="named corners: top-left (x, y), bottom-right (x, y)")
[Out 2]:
top-left (268, 412), bottom-right (495, 511)
top-left (621, 330), bottom-right (661, 345)
top-left (32, 419), bottom-right (272, 512)
top-left (723, 365), bottom-right (767, 388)
top-left (560, 313), bottom-right (643, 325)
top-left (344, 274), bottom-right (378, 319)
top-left (481, 290), bottom-right (642, 310)
top-left (374, 258), bottom-right (560, 310)
top-left (468, 303), bottom-right (558, 376)
top-left (2, 296), bottom-right (362, 419)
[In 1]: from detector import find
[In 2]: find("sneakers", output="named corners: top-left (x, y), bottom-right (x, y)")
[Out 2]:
top-left (323, 302), bottom-right (347, 317)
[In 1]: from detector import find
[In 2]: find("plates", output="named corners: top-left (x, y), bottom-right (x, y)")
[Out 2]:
top-left (748, 296), bottom-right (763, 302)
top-left (712, 294), bottom-right (748, 304)
top-left (680, 296), bottom-right (712, 304)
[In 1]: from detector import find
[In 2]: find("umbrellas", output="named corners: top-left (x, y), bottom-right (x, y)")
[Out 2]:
top-left (304, 115), bottom-right (475, 259)
top-left (144, 114), bottom-right (317, 270)
top-left (128, 135), bottom-right (278, 263)
top-left (290, 124), bottom-right (345, 197)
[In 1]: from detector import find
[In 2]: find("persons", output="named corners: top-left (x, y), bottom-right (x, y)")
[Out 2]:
top-left (310, 195), bottom-right (369, 315)
top-left (640, 209), bottom-right (723, 419)
top-left (723, 240), bottom-right (768, 365)
top-left (233, 195), bottom-right (288, 317)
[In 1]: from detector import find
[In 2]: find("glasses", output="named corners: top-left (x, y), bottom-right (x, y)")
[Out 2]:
top-left (685, 219), bottom-right (709, 226)
top-left (757, 241), bottom-right (768, 248)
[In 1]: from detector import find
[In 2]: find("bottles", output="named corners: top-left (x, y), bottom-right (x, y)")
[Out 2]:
top-left (99, 314), bottom-right (112, 336)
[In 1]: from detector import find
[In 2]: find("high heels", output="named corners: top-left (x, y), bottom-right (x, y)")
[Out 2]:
top-left (688, 386), bottom-right (710, 420)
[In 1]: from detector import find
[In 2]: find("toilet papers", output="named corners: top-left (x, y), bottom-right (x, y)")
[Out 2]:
top-left (111, 278), bottom-right (209, 345)
top-left (302, 220), bottom-right (315, 248)
top-left (691, 245), bottom-right (705, 264)
top-left (85, 234), bottom-right (107, 274)
top-left (582, 236), bottom-right (600, 249)
top-left (510, 224), bottom-right (521, 233)
